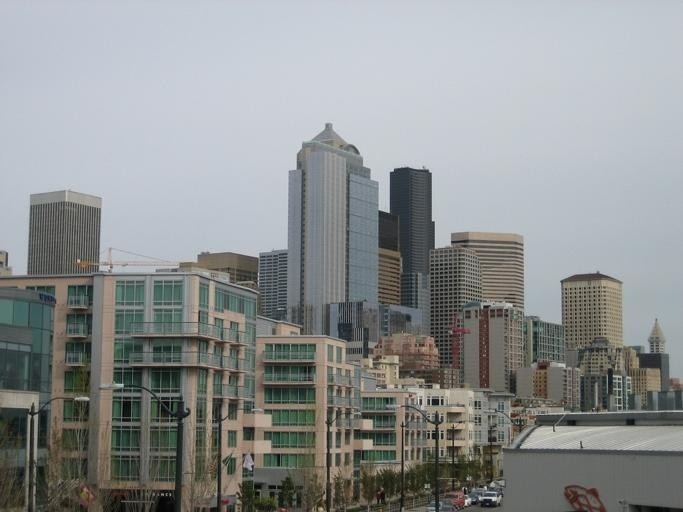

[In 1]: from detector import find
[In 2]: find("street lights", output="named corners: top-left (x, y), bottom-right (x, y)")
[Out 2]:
top-left (490, 409), bottom-right (524, 433)
top-left (213, 402), bottom-right (265, 512)
top-left (401, 403), bottom-right (445, 512)
top-left (490, 422), bottom-right (498, 483)
top-left (325, 409), bottom-right (362, 512)
top-left (24, 392), bottom-right (92, 512)
top-left (98, 380), bottom-right (193, 512)
top-left (450, 420), bottom-right (465, 492)
top-left (400, 417), bottom-right (428, 512)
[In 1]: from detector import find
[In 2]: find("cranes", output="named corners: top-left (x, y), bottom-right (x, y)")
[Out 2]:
top-left (448, 315), bottom-right (471, 368)
top-left (73, 245), bottom-right (187, 273)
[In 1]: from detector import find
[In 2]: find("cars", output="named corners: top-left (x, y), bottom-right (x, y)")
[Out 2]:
top-left (426, 477), bottom-right (503, 512)
